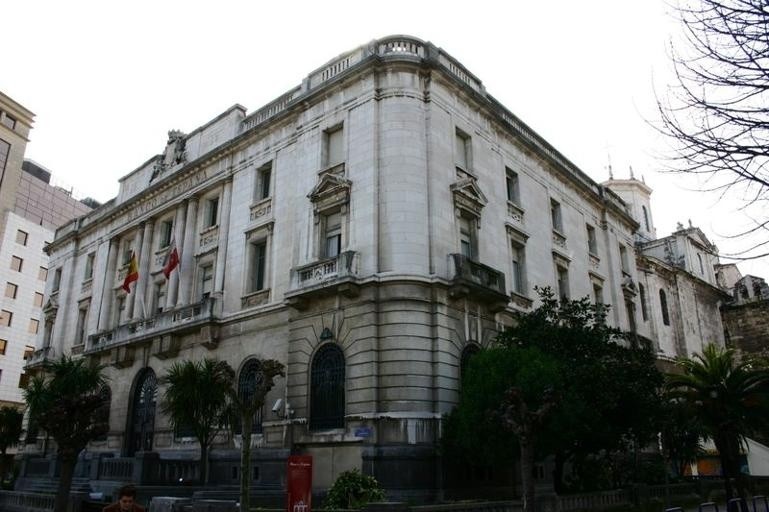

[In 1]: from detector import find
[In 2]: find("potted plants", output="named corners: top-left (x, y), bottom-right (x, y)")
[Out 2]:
top-left (316, 467), bottom-right (390, 509)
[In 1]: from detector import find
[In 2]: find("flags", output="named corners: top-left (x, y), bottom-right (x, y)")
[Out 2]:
top-left (122, 251), bottom-right (138, 293)
top-left (161, 238), bottom-right (180, 280)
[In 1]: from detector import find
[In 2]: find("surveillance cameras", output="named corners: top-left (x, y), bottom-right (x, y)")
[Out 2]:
top-left (271, 399), bottom-right (283, 413)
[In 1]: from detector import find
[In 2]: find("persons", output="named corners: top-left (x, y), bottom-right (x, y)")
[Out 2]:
top-left (102, 485), bottom-right (146, 512)
